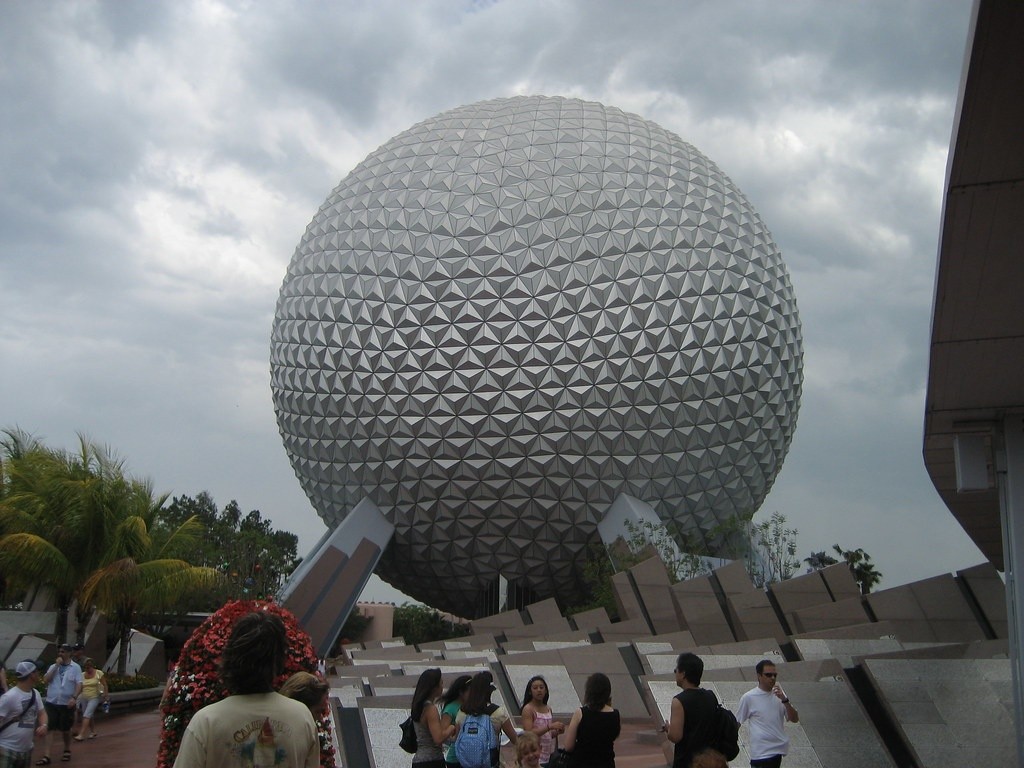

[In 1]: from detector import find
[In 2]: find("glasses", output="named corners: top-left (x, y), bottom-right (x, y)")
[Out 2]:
top-left (465, 679), bottom-right (472, 684)
top-left (73, 648), bottom-right (81, 651)
top-left (58, 651), bottom-right (64, 653)
top-left (674, 669), bottom-right (676, 673)
top-left (760, 672), bottom-right (778, 677)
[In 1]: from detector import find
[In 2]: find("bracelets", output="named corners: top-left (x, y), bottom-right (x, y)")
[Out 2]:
top-left (43, 723), bottom-right (48, 726)
top-left (782, 698), bottom-right (789, 703)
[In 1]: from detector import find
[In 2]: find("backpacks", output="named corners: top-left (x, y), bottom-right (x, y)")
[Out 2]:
top-left (455, 712), bottom-right (497, 768)
top-left (706, 707), bottom-right (740, 761)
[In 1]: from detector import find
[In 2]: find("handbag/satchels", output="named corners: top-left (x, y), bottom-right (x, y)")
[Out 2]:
top-left (102, 696), bottom-right (112, 713)
top-left (399, 716), bottom-right (418, 753)
top-left (548, 749), bottom-right (570, 768)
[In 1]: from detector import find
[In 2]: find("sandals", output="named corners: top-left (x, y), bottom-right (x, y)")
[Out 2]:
top-left (61, 750), bottom-right (72, 761)
top-left (35, 755), bottom-right (51, 765)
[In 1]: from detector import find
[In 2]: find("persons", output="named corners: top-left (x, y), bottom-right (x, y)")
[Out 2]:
top-left (172, 610), bottom-right (330, 768)
top-left (519, 676), bottom-right (565, 768)
top-left (563, 673), bottom-right (621, 768)
top-left (411, 668), bottom-right (517, 768)
top-left (516, 730), bottom-right (544, 768)
top-left (35, 643), bottom-right (108, 768)
top-left (0, 658), bottom-right (48, 768)
top-left (735, 660), bottom-right (799, 768)
top-left (656, 652), bottom-right (739, 768)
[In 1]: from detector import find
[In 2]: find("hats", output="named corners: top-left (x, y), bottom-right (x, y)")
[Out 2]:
top-left (72, 643), bottom-right (85, 650)
top-left (15, 658), bottom-right (45, 679)
top-left (57, 643), bottom-right (72, 651)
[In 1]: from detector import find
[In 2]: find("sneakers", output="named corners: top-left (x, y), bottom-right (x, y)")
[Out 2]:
top-left (88, 733), bottom-right (97, 738)
top-left (74, 735), bottom-right (85, 740)
